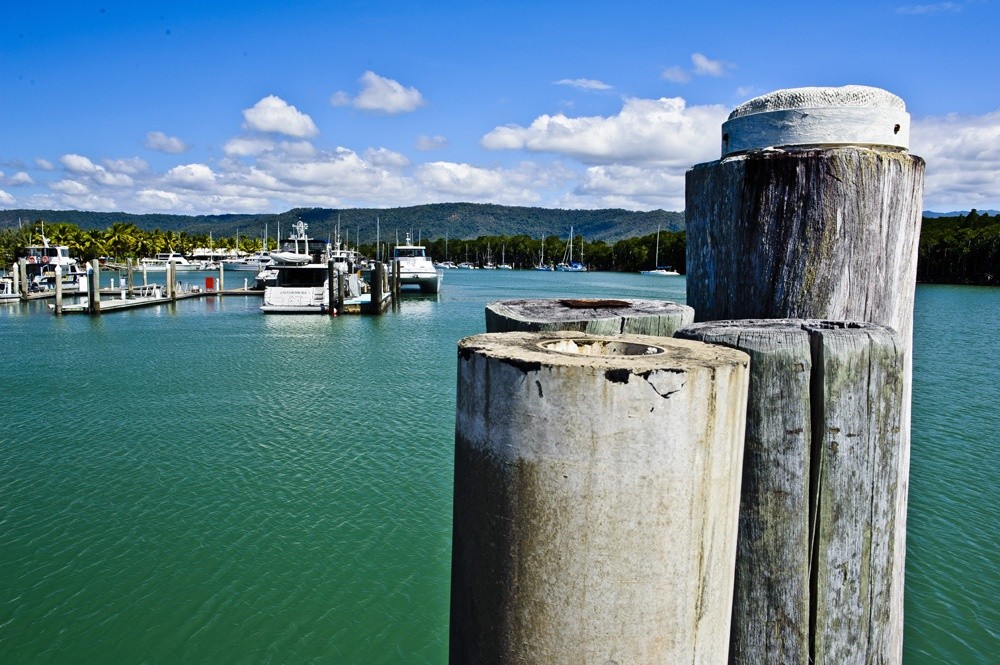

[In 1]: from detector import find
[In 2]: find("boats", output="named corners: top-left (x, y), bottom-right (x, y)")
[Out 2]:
top-left (0, 221), bottom-right (87, 292)
top-left (388, 225), bottom-right (443, 294)
top-left (135, 211), bottom-right (367, 312)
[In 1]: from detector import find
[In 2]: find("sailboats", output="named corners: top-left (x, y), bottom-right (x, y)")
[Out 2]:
top-left (432, 230), bottom-right (513, 270)
top-left (533, 225), bottom-right (587, 272)
top-left (640, 222), bottom-right (680, 277)
